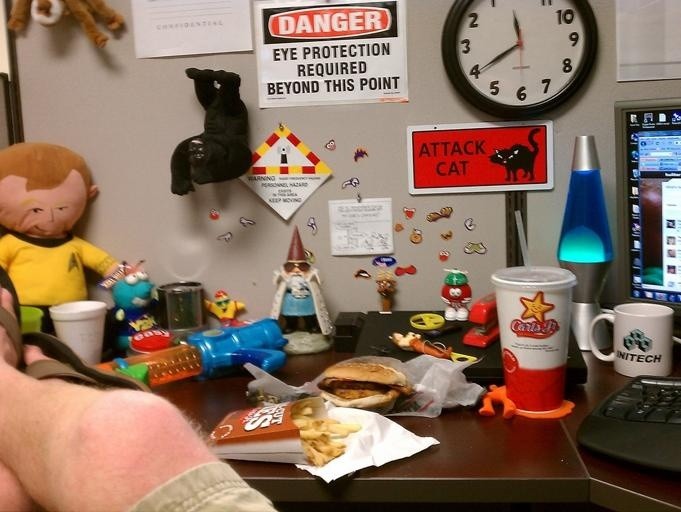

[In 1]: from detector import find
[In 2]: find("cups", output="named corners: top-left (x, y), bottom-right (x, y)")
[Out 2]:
top-left (19, 307), bottom-right (47, 335)
top-left (153, 282), bottom-right (205, 337)
top-left (491, 266), bottom-right (577, 418)
top-left (49, 298), bottom-right (107, 367)
top-left (589, 302), bottom-right (674, 378)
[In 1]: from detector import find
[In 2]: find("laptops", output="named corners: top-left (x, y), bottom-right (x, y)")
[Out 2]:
top-left (356, 311), bottom-right (586, 386)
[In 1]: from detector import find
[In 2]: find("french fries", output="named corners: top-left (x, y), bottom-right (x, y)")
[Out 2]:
top-left (291, 399), bottom-right (358, 468)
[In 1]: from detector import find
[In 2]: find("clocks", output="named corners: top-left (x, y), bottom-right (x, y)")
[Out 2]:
top-left (441, 0), bottom-right (598, 119)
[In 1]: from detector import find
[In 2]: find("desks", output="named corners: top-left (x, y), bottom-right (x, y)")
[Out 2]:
top-left (117, 328), bottom-right (681, 512)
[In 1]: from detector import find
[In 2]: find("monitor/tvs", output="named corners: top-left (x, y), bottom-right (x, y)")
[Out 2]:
top-left (614, 97), bottom-right (681, 335)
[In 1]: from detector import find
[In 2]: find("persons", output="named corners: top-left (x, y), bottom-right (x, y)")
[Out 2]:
top-left (375, 262), bottom-right (515, 421)
top-left (1, 142), bottom-right (125, 355)
top-left (1, 264), bottom-right (277, 512)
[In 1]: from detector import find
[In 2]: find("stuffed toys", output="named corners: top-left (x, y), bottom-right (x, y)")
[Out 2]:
top-left (168, 67), bottom-right (252, 198)
top-left (8, 1), bottom-right (126, 52)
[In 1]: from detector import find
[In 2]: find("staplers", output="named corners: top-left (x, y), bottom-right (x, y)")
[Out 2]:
top-left (462, 291), bottom-right (500, 348)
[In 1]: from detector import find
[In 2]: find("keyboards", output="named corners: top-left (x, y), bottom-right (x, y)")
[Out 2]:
top-left (578, 375), bottom-right (681, 473)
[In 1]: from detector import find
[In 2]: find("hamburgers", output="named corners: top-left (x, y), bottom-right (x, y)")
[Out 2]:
top-left (316, 360), bottom-right (414, 415)
